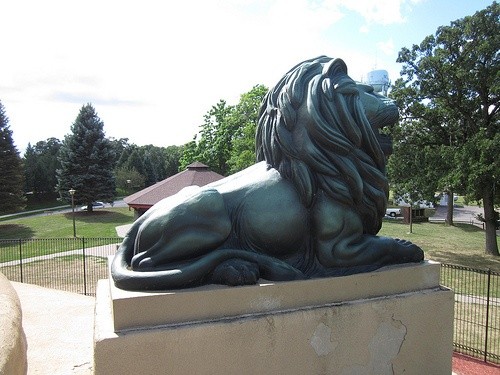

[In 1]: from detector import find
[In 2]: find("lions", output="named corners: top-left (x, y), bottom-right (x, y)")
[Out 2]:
top-left (111, 56), bottom-right (425, 291)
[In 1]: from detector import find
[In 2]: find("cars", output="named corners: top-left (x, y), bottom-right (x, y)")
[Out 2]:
top-left (435, 191), bottom-right (458, 201)
top-left (81, 202), bottom-right (105, 210)
top-left (385, 191), bottom-right (434, 217)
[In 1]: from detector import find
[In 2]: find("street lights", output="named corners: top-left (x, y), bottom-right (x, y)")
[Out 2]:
top-left (126, 179), bottom-right (131, 212)
top-left (69, 188), bottom-right (76, 237)
